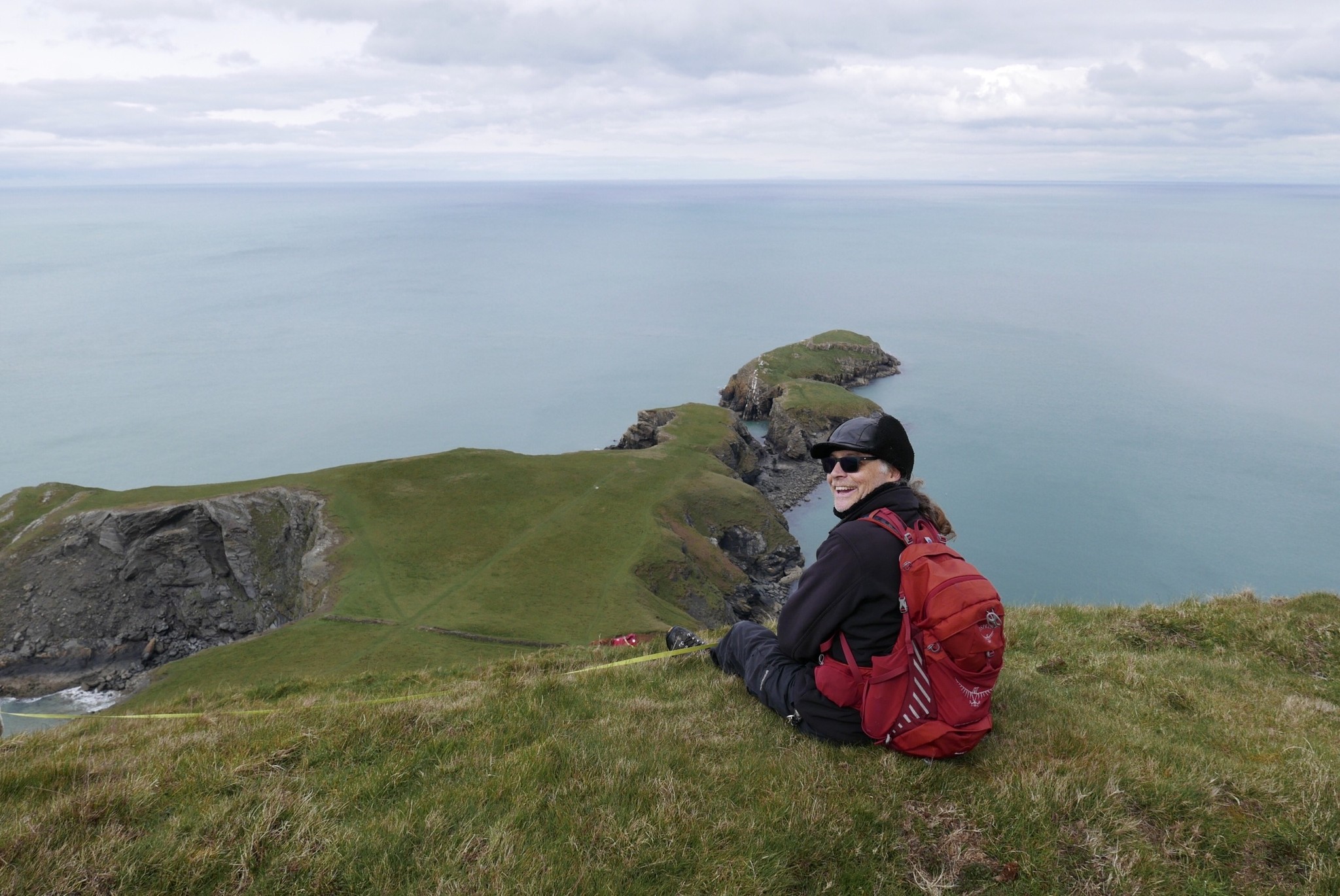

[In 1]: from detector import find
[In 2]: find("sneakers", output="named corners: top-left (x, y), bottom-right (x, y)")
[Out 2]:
top-left (665, 625), bottom-right (705, 657)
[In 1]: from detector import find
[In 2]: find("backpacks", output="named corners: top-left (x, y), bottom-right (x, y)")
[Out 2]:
top-left (813, 507), bottom-right (1006, 759)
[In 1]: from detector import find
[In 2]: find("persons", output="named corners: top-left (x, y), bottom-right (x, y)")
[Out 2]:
top-left (666, 414), bottom-right (956, 746)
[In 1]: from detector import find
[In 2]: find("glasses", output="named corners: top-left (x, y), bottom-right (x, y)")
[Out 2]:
top-left (821, 456), bottom-right (879, 473)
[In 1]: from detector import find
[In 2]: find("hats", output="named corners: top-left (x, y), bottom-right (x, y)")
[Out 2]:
top-left (809, 409), bottom-right (914, 482)
